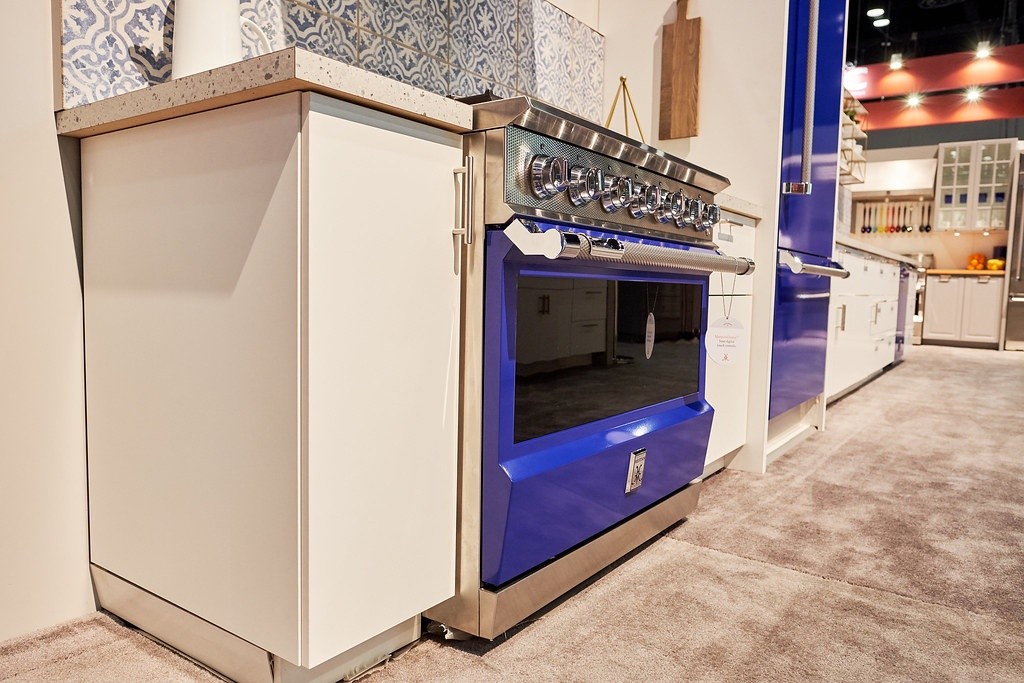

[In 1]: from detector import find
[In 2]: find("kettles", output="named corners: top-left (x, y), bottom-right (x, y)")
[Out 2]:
top-left (172, 1), bottom-right (272, 83)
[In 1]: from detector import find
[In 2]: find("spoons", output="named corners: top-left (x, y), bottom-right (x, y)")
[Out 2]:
top-left (885, 205), bottom-right (889, 233)
top-left (907, 204), bottom-right (913, 232)
top-left (896, 206), bottom-right (900, 232)
top-left (866, 202), bottom-right (871, 233)
top-left (861, 203), bottom-right (866, 233)
top-left (902, 205), bottom-right (907, 232)
top-left (872, 203), bottom-right (877, 233)
top-left (878, 204), bottom-right (883, 233)
top-left (925, 203), bottom-right (931, 232)
top-left (890, 205), bottom-right (895, 232)
top-left (919, 206), bottom-right (925, 233)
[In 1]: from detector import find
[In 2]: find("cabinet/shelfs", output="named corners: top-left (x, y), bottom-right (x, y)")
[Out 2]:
top-left (704, 210), bottom-right (756, 480)
top-left (825, 243), bottom-right (901, 407)
top-left (922, 275), bottom-right (1003, 343)
top-left (932, 137), bottom-right (1018, 232)
top-left (840, 88), bottom-right (870, 186)
top-left (80, 89), bottom-right (476, 671)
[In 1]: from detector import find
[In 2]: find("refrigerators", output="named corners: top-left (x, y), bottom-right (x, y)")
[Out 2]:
top-left (604, 1), bottom-right (849, 472)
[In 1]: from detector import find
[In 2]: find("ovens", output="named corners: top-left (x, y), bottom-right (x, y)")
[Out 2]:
top-left (420, 96), bottom-right (757, 643)
